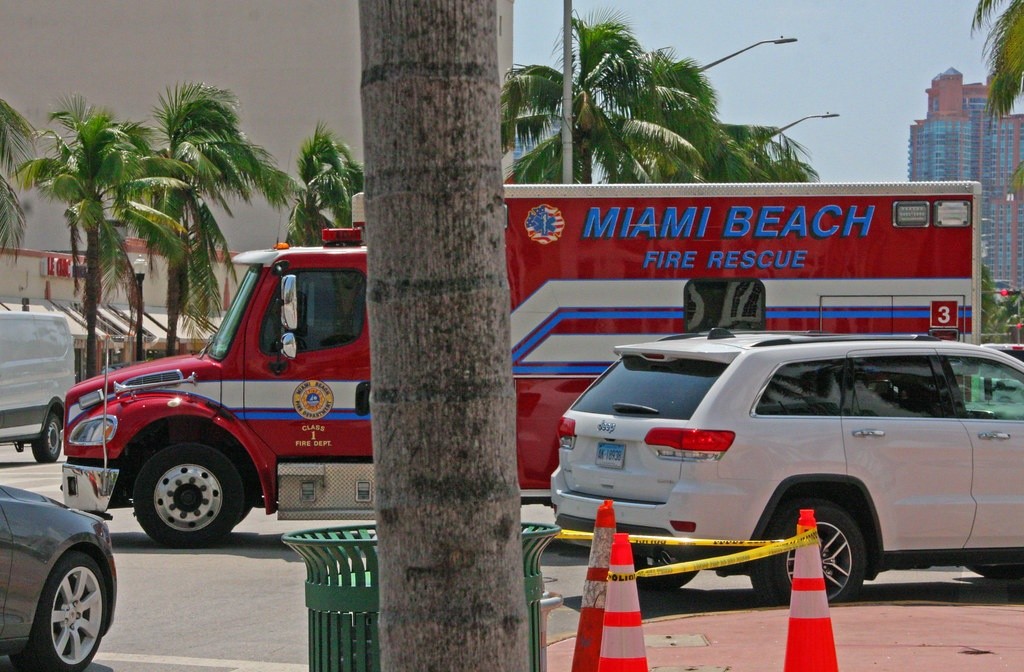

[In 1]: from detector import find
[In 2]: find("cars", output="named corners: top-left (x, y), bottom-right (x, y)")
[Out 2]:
top-left (0, 485), bottom-right (118, 672)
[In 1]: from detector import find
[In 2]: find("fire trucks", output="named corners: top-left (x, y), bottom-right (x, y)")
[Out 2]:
top-left (62, 180), bottom-right (982, 550)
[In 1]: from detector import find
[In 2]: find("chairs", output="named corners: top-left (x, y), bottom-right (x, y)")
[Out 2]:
top-left (855, 369), bottom-right (945, 417)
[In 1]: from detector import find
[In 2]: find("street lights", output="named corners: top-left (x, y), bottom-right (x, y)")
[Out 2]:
top-left (697, 38), bottom-right (798, 72)
top-left (770, 113), bottom-right (841, 136)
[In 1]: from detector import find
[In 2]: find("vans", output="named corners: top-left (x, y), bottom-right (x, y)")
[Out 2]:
top-left (1, 312), bottom-right (74, 464)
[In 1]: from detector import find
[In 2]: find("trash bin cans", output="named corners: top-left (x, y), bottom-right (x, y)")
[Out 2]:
top-left (279, 521), bottom-right (565, 672)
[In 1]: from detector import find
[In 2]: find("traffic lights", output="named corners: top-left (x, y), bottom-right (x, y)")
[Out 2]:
top-left (1001, 289), bottom-right (1021, 296)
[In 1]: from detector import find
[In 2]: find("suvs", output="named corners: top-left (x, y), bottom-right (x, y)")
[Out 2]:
top-left (550, 325), bottom-right (1024, 608)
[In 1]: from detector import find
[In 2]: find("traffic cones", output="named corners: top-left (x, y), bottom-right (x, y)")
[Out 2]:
top-left (784, 510), bottom-right (839, 672)
top-left (598, 534), bottom-right (648, 672)
top-left (570, 500), bottom-right (616, 672)
